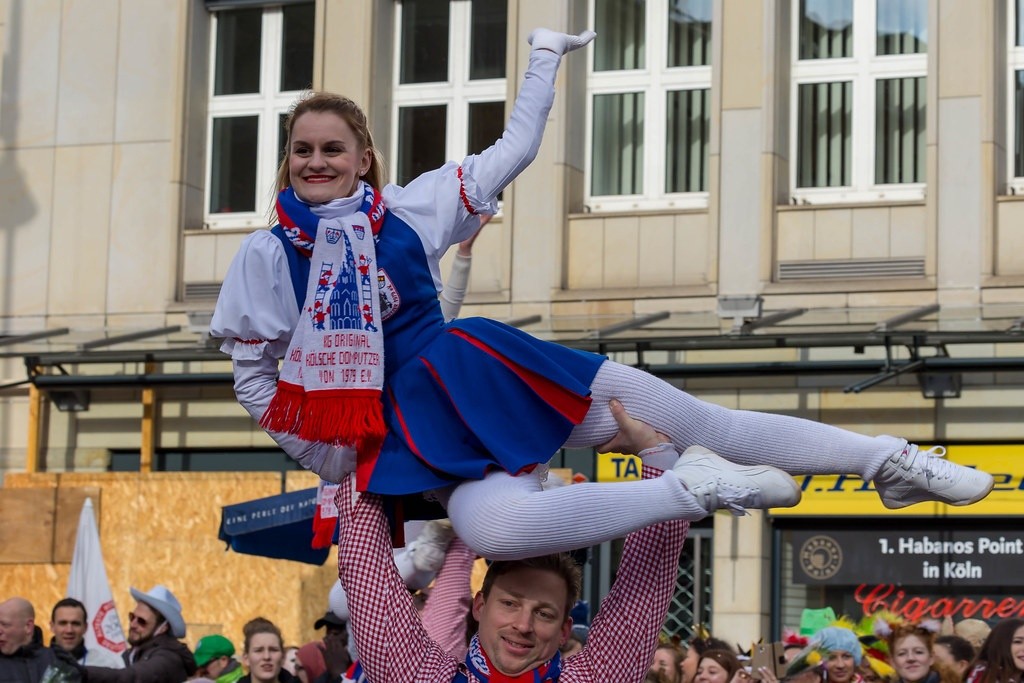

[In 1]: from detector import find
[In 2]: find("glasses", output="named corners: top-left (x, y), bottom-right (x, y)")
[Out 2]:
top-left (129, 612), bottom-right (160, 629)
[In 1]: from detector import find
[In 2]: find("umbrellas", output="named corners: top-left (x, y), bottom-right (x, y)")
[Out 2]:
top-left (65, 497), bottom-right (126, 668)
top-left (220, 481), bottom-right (340, 568)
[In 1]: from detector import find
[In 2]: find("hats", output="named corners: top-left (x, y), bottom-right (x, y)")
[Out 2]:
top-left (192, 635), bottom-right (235, 667)
top-left (128, 586), bottom-right (186, 639)
top-left (314, 612), bottom-right (347, 630)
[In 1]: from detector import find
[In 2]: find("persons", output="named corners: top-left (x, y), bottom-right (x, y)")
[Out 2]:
top-left (643, 607), bottom-right (1024, 683)
top-left (332, 399), bottom-right (691, 683)
top-left (206, 23), bottom-right (998, 562)
top-left (1, 521), bottom-right (474, 683)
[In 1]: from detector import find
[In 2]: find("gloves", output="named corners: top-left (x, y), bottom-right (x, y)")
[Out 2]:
top-left (330, 445), bottom-right (357, 484)
top-left (528, 28), bottom-right (597, 57)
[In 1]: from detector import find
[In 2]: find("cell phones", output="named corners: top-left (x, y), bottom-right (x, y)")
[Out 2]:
top-left (752, 642), bottom-right (776, 680)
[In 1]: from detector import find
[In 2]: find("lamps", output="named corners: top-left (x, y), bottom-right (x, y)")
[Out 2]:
top-left (718, 294), bottom-right (763, 335)
top-left (188, 312), bottom-right (216, 347)
top-left (47, 388), bottom-right (91, 411)
top-left (919, 372), bottom-right (962, 397)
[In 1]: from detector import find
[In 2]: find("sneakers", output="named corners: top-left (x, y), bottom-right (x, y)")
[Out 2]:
top-left (872, 440), bottom-right (995, 510)
top-left (669, 443), bottom-right (803, 517)
